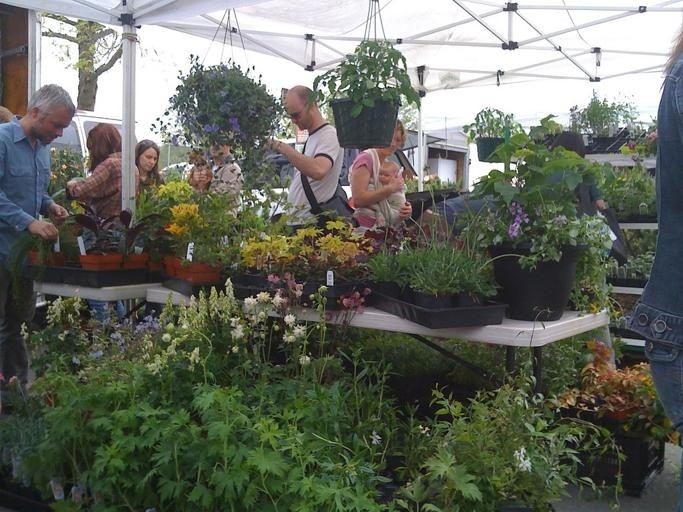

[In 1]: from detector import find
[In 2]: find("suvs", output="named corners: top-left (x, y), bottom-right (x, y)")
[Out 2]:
top-left (155, 134), bottom-right (364, 225)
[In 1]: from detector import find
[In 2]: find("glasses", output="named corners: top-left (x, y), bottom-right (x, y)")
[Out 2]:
top-left (291, 104), bottom-right (308, 120)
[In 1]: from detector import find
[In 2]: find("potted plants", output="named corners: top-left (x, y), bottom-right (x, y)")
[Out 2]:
top-left (570, 89), bottom-right (640, 154)
top-left (463, 106), bottom-right (526, 163)
top-left (302, 40), bottom-right (422, 150)
top-left (364, 163), bottom-right (498, 308)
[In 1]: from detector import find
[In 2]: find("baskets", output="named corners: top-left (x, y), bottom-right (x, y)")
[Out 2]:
top-left (561, 434), bottom-right (665, 490)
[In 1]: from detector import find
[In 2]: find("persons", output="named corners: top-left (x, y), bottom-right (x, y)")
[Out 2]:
top-left (377, 161), bottom-right (406, 228)
top-left (133, 136), bottom-right (164, 208)
top-left (0, 82), bottom-right (77, 383)
top-left (264, 85), bottom-right (345, 231)
top-left (47, 121), bottom-right (142, 368)
top-left (621, 27), bottom-right (683, 435)
top-left (184, 140), bottom-right (241, 216)
top-left (575, 176), bottom-right (611, 262)
top-left (186, 149), bottom-right (210, 193)
top-left (348, 116), bottom-right (414, 233)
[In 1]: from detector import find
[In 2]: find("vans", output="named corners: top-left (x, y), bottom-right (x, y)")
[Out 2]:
top-left (44, 108), bottom-right (136, 178)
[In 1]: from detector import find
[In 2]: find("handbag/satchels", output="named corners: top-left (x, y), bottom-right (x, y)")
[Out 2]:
top-left (597, 209), bottom-right (628, 266)
top-left (311, 184), bottom-right (358, 231)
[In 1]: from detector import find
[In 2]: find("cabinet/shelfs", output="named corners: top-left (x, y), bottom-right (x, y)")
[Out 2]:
top-left (586, 148), bottom-right (657, 347)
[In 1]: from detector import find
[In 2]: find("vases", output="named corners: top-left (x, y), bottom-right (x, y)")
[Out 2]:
top-left (488, 241), bottom-right (590, 322)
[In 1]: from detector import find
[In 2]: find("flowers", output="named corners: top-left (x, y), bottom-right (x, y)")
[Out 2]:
top-left (484, 201), bottom-right (614, 285)
top-left (149, 51), bottom-right (300, 170)
